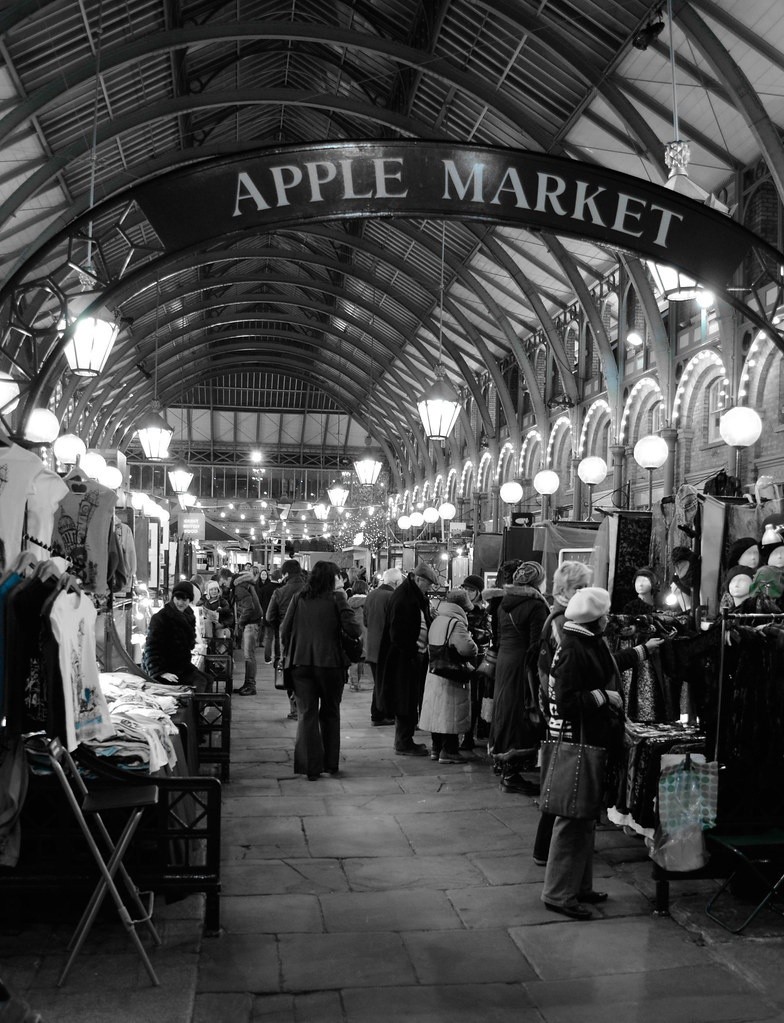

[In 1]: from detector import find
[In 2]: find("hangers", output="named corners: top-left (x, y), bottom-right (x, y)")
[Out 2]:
top-left (66, 453), bottom-right (89, 494)
top-left (0, 418), bottom-right (12, 447)
top-left (11, 534), bottom-right (81, 596)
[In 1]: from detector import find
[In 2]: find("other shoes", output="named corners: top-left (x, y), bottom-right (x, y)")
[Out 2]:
top-left (394, 739), bottom-right (430, 757)
top-left (306, 771), bottom-right (320, 782)
top-left (264, 660), bottom-right (273, 664)
top-left (430, 749), bottom-right (468, 764)
top-left (499, 774), bottom-right (535, 792)
top-left (233, 686), bottom-right (257, 695)
top-left (350, 683), bottom-right (362, 692)
top-left (369, 717), bottom-right (396, 726)
top-left (545, 889), bottom-right (610, 919)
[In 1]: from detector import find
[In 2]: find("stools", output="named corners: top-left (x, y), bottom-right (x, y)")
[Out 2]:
top-left (46, 736), bottom-right (162, 991)
top-left (704, 831), bottom-right (784, 934)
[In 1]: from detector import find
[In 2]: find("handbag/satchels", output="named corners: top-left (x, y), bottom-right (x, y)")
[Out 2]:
top-left (427, 617), bottom-right (473, 681)
top-left (657, 757), bottom-right (721, 829)
top-left (539, 717), bottom-right (611, 819)
top-left (476, 650), bottom-right (498, 677)
top-left (337, 627), bottom-right (364, 663)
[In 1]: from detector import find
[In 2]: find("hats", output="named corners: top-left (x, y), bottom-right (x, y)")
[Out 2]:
top-left (460, 575), bottom-right (485, 592)
top-left (565, 587), bottom-right (610, 623)
top-left (513, 561), bottom-right (545, 586)
top-left (205, 580), bottom-right (221, 600)
top-left (172, 581), bottom-right (194, 602)
top-left (412, 562), bottom-right (439, 585)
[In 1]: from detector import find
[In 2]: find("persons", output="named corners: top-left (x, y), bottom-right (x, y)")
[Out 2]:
top-left (140, 581), bottom-right (214, 742)
top-left (192, 553), bottom-right (668, 918)
top-left (623, 514), bottom-right (783, 616)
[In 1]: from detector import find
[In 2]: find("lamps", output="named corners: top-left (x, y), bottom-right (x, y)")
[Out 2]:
top-left (136, 250), bottom-right (197, 512)
top-left (646, 0), bottom-right (729, 302)
top-left (0, 370), bottom-right (171, 523)
top-left (314, 252), bottom-right (384, 520)
top-left (397, 406), bottom-right (762, 542)
top-left (55, 0), bottom-right (123, 379)
top-left (417, 102), bottom-right (464, 442)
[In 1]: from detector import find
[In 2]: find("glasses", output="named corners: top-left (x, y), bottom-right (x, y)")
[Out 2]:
top-left (175, 596), bottom-right (187, 601)
